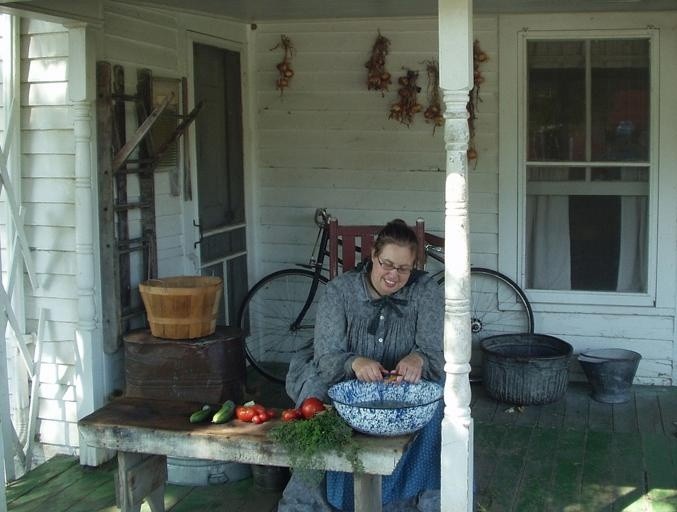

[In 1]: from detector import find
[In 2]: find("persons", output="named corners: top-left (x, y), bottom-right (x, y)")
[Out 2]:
top-left (284, 218), bottom-right (445, 510)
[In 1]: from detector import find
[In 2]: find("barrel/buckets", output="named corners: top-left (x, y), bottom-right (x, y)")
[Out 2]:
top-left (123, 326), bottom-right (251, 483)
top-left (137, 276), bottom-right (224, 340)
top-left (575, 347), bottom-right (643, 404)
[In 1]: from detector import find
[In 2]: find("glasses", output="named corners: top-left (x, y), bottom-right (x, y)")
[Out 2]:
top-left (377, 255), bottom-right (410, 274)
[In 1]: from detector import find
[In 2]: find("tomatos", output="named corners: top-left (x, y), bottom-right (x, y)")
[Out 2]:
top-left (238, 404), bottom-right (275, 424)
top-left (282, 409), bottom-right (300, 420)
top-left (302, 397), bottom-right (322, 418)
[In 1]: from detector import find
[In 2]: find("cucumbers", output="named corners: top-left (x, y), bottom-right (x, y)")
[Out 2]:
top-left (212, 400), bottom-right (234, 423)
top-left (190, 405), bottom-right (211, 422)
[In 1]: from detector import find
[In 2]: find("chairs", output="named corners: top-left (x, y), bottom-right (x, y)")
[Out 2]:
top-left (326, 213), bottom-right (427, 283)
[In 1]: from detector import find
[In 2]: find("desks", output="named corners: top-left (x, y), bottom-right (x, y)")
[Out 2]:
top-left (75, 396), bottom-right (423, 512)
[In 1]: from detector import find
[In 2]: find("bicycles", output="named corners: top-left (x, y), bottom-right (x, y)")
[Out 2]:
top-left (237, 208), bottom-right (534, 386)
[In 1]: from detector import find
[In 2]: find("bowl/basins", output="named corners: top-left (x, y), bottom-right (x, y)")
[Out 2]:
top-left (326, 377), bottom-right (442, 436)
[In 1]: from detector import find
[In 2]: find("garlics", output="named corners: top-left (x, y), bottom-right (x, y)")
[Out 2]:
top-left (365, 41), bottom-right (438, 119)
top-left (276, 61), bottom-right (293, 87)
top-left (468, 150), bottom-right (476, 159)
top-left (474, 48), bottom-right (486, 84)
